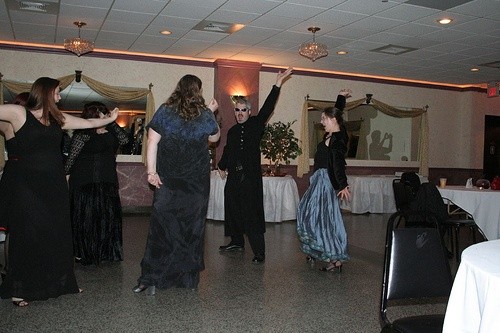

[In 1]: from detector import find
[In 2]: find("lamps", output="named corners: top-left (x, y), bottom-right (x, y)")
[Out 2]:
top-left (64, 22), bottom-right (95, 57)
top-left (298, 26), bottom-right (328, 61)
top-left (229, 95), bottom-right (246, 104)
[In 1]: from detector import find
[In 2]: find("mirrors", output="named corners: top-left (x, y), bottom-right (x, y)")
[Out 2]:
top-left (0, 82), bottom-right (147, 162)
top-left (306, 100), bottom-right (422, 161)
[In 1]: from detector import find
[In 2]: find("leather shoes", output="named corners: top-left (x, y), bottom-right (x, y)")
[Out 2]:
top-left (219, 242), bottom-right (243, 251)
top-left (251, 256), bottom-right (264, 262)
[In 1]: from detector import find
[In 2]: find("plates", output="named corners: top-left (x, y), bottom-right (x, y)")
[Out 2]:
top-left (274, 173), bottom-right (288, 176)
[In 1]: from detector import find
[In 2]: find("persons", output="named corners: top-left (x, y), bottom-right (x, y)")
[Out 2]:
top-left (134, 74), bottom-right (220, 295)
top-left (217, 65), bottom-right (293, 264)
top-left (65, 101), bottom-right (129, 265)
top-left (297, 87), bottom-right (354, 273)
top-left (0, 77), bottom-right (120, 304)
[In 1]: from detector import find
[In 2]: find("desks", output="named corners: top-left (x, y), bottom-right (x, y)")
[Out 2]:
top-left (442, 239), bottom-right (500, 333)
top-left (205, 170), bottom-right (301, 223)
top-left (435, 185), bottom-right (500, 241)
top-left (340, 175), bottom-right (430, 214)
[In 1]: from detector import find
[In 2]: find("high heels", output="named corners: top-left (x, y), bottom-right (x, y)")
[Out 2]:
top-left (305, 254), bottom-right (315, 263)
top-left (319, 259), bottom-right (342, 274)
top-left (133, 277), bottom-right (156, 295)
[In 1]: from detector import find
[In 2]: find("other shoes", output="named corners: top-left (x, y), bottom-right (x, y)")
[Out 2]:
top-left (11, 297), bottom-right (28, 306)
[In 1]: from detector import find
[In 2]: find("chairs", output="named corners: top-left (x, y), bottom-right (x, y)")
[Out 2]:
top-left (379, 171), bottom-right (488, 333)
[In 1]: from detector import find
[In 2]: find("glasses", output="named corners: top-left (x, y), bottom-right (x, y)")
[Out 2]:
top-left (233, 107), bottom-right (249, 112)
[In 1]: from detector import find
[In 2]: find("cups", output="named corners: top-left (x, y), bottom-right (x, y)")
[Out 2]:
top-left (440, 178), bottom-right (446, 187)
